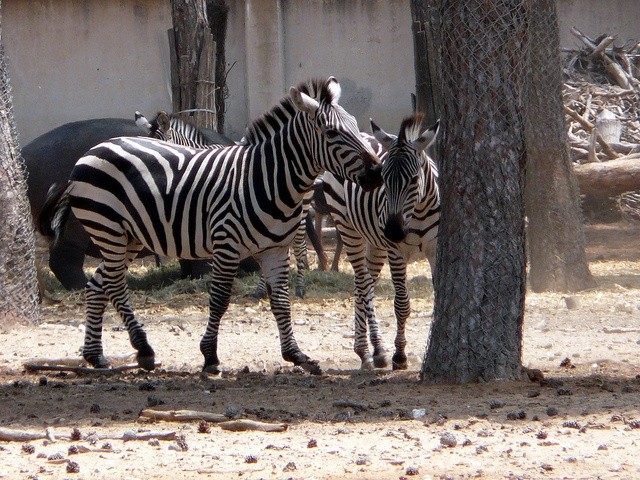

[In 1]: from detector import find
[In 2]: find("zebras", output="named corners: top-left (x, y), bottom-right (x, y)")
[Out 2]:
top-left (133, 109), bottom-right (323, 301)
top-left (321, 111), bottom-right (443, 372)
top-left (34, 78), bottom-right (387, 377)
